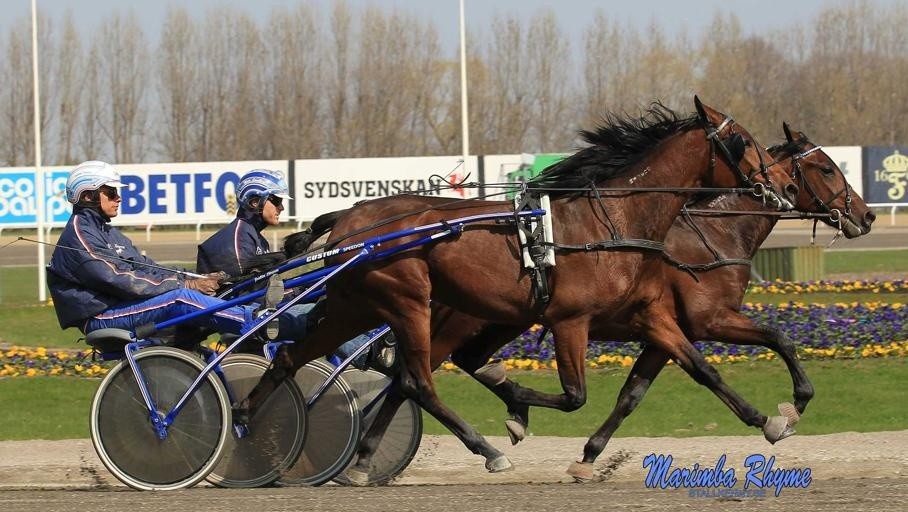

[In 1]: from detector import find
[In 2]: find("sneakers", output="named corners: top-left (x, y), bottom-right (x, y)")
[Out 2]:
top-left (253, 274), bottom-right (284, 341)
top-left (370, 333), bottom-right (396, 365)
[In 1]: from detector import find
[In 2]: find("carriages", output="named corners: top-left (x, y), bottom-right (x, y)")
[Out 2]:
top-left (81, 88), bottom-right (876, 497)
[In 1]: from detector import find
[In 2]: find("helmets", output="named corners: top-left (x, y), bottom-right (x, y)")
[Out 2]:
top-left (236, 170), bottom-right (293, 209)
top-left (66, 161), bottom-right (128, 204)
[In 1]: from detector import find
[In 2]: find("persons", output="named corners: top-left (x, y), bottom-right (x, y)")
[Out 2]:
top-left (46, 162), bottom-right (286, 372)
top-left (197, 168), bottom-right (398, 374)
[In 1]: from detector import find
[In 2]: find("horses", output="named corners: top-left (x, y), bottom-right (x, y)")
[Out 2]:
top-left (340, 119), bottom-right (876, 490)
top-left (226, 95), bottom-right (804, 475)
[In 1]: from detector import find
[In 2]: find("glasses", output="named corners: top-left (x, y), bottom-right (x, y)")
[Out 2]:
top-left (268, 196), bottom-right (282, 206)
top-left (101, 188), bottom-right (117, 199)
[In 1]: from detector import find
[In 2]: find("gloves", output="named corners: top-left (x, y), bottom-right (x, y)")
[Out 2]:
top-left (186, 271), bottom-right (220, 295)
